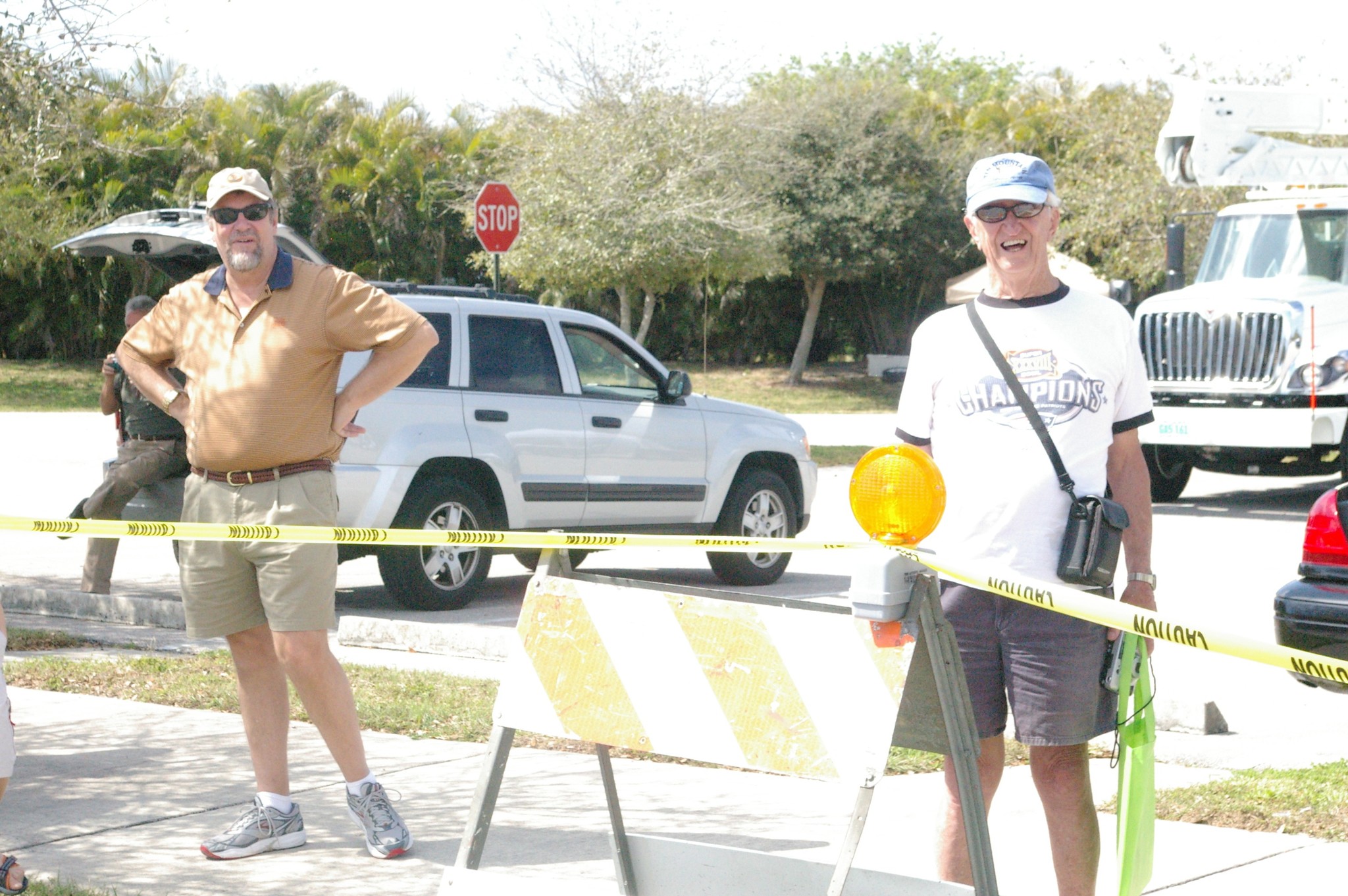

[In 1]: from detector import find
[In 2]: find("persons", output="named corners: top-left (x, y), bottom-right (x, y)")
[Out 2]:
top-left (116, 167), bottom-right (439, 859)
top-left (896, 152), bottom-right (1159, 896)
top-left (58, 294), bottom-right (188, 594)
top-left (0, 605), bottom-right (31, 895)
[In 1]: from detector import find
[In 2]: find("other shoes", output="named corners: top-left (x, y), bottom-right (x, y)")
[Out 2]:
top-left (56, 498), bottom-right (88, 540)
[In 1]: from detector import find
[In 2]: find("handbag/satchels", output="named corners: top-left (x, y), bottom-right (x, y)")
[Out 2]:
top-left (1058, 495), bottom-right (1129, 589)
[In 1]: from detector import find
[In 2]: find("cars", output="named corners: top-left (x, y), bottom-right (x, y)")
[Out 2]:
top-left (1272, 485), bottom-right (1348, 694)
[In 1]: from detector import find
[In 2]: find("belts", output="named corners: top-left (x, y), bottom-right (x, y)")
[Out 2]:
top-left (129, 434), bottom-right (186, 441)
top-left (190, 460), bottom-right (331, 487)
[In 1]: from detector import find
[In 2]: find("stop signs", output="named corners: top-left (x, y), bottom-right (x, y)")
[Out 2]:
top-left (474, 181), bottom-right (521, 253)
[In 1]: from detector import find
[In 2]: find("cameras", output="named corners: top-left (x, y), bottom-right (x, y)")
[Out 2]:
top-left (1101, 628), bottom-right (1142, 696)
top-left (108, 359), bottom-right (122, 372)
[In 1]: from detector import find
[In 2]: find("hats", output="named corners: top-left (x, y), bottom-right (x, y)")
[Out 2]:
top-left (207, 167), bottom-right (273, 210)
top-left (966, 153), bottom-right (1056, 215)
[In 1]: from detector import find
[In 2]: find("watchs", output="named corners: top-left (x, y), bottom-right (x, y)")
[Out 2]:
top-left (1126, 573), bottom-right (1157, 591)
top-left (162, 387), bottom-right (187, 417)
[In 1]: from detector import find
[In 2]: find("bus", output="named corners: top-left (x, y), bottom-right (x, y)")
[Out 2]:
top-left (1132, 190), bottom-right (1348, 504)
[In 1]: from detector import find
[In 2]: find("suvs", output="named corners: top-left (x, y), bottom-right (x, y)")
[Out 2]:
top-left (50, 205), bottom-right (820, 613)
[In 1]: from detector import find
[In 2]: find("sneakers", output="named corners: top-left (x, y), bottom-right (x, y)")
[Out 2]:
top-left (201, 796), bottom-right (306, 859)
top-left (345, 782), bottom-right (412, 859)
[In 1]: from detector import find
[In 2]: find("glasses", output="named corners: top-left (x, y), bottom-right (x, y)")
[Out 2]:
top-left (209, 203), bottom-right (269, 224)
top-left (975, 202), bottom-right (1045, 223)
